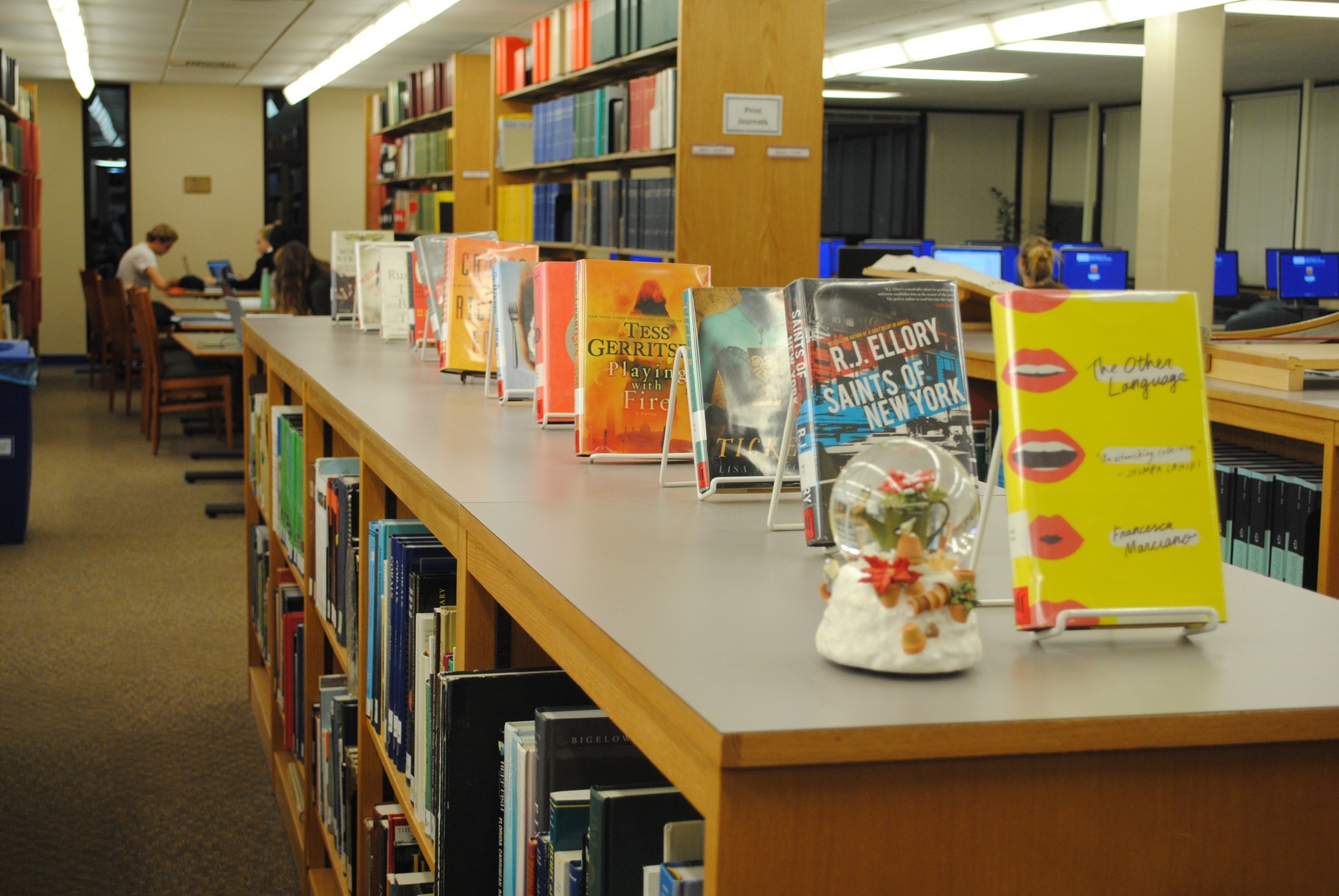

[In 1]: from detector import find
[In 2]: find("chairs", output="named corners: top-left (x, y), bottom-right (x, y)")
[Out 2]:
top-left (133, 287), bottom-right (235, 458)
top-left (76, 267), bottom-right (142, 394)
top-left (105, 275), bottom-right (179, 419)
top-left (125, 283), bottom-right (227, 439)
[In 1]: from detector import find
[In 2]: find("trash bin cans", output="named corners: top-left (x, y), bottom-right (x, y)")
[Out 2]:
top-left (1, 339), bottom-right (38, 544)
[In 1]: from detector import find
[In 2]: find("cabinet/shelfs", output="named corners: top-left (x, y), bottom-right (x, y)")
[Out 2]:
top-left (238, 0), bottom-right (1339, 896)
top-left (0, 48), bottom-right (46, 383)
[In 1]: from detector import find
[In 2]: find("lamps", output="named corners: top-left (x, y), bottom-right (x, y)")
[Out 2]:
top-left (899, 14), bottom-right (998, 63)
top-left (988, 0), bottom-right (1121, 47)
top-left (1099, 0), bottom-right (1248, 29)
top-left (822, 50), bottom-right (837, 80)
top-left (828, 37), bottom-right (913, 77)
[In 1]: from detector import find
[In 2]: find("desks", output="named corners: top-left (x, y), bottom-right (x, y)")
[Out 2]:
top-left (146, 284), bottom-right (293, 462)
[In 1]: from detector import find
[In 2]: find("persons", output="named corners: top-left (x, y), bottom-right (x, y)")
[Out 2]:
top-left (115, 223), bottom-right (181, 352)
top-left (222, 223), bottom-right (283, 291)
top-left (273, 238), bottom-right (329, 316)
top-left (1014, 233), bottom-right (1067, 288)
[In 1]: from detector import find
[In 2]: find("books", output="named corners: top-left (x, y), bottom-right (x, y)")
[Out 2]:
top-left (0, 50), bottom-right (43, 340)
top-left (378, 128), bottom-right (451, 178)
top-left (329, 230), bottom-right (500, 342)
top-left (784, 275), bottom-right (979, 551)
top-left (574, 259), bottom-right (711, 463)
top-left (497, 66), bottom-right (676, 167)
top-left (990, 288), bottom-right (1229, 631)
top-left (1211, 444), bottom-right (1322, 588)
top-left (378, 188), bottom-right (453, 232)
top-left (248, 388), bottom-right (702, 896)
top-left (494, 0), bottom-right (678, 96)
top-left (493, 261), bottom-right (574, 425)
top-left (493, 177), bottom-right (675, 251)
top-left (431, 237), bottom-right (540, 378)
top-left (683, 287), bottom-right (802, 499)
top-left (369, 60), bottom-right (451, 131)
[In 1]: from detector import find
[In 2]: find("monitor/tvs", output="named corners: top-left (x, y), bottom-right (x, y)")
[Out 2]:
top-left (1277, 251), bottom-right (1339, 299)
top-left (1266, 249), bottom-right (1321, 290)
top-left (1058, 247), bottom-right (1128, 291)
top-left (819, 237), bottom-right (1024, 286)
top-left (1215, 251), bottom-right (1239, 296)
top-left (1051, 241), bottom-right (1101, 281)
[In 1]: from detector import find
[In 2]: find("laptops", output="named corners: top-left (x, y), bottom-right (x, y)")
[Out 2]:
top-left (206, 259), bottom-right (233, 285)
top-left (225, 297), bottom-right (249, 342)
